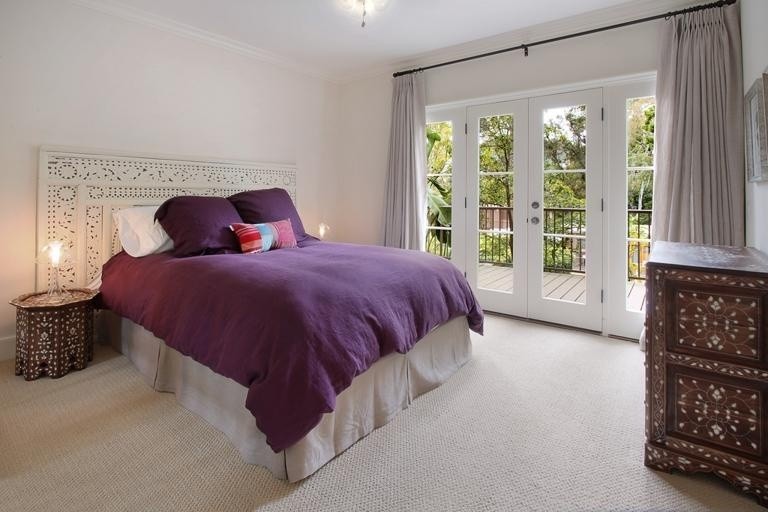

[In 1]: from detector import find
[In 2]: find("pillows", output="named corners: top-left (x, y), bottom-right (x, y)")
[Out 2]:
top-left (112, 206), bottom-right (174, 257)
top-left (154, 195), bottom-right (245, 258)
top-left (230, 218), bottom-right (300, 256)
top-left (227, 188), bottom-right (309, 243)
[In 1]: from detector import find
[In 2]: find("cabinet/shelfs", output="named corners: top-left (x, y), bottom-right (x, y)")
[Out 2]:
top-left (644, 240), bottom-right (768, 509)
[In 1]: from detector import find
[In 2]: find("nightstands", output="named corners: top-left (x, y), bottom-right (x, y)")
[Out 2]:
top-left (9, 287), bottom-right (101, 381)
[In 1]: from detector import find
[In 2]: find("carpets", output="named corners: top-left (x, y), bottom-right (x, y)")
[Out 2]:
top-left (0, 312), bottom-right (768, 512)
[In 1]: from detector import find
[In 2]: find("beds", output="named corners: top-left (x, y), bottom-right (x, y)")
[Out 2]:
top-left (36, 143), bottom-right (483, 484)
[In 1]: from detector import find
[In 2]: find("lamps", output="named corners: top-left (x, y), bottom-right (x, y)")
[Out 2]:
top-left (46, 240), bottom-right (72, 301)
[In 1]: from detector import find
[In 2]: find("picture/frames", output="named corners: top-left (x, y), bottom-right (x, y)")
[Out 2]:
top-left (744, 72), bottom-right (768, 183)
